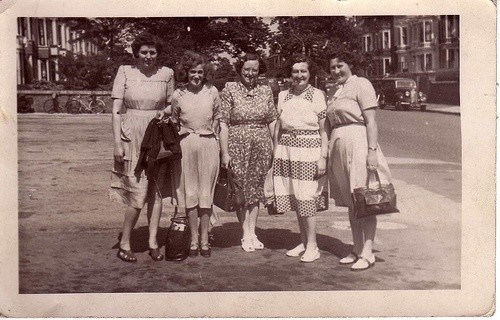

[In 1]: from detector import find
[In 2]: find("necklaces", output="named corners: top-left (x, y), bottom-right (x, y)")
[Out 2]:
top-left (292, 84), bottom-right (310, 92)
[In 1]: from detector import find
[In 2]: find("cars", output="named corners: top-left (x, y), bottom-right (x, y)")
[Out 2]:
top-left (372, 77), bottom-right (428, 112)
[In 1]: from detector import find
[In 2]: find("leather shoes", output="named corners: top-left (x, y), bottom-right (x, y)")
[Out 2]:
top-left (189, 242), bottom-right (200, 257)
top-left (199, 241), bottom-right (211, 258)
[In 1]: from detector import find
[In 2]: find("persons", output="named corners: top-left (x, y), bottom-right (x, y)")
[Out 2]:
top-left (323, 50), bottom-right (394, 270)
top-left (164, 50), bottom-right (221, 258)
top-left (261, 54), bottom-right (330, 262)
top-left (219, 53), bottom-right (278, 253)
top-left (110, 32), bottom-right (176, 262)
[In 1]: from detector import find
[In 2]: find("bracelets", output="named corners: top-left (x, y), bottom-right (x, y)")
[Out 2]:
top-left (368, 146), bottom-right (377, 151)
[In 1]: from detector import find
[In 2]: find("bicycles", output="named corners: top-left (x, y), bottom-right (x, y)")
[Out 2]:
top-left (42, 92), bottom-right (105, 115)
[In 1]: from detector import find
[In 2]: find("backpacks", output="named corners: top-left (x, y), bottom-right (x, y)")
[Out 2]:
top-left (141, 118), bottom-right (182, 166)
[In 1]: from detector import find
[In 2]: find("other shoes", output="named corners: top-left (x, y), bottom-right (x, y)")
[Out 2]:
top-left (286, 243), bottom-right (307, 256)
top-left (351, 253), bottom-right (375, 270)
top-left (251, 235), bottom-right (264, 250)
top-left (300, 247), bottom-right (321, 262)
top-left (340, 251), bottom-right (361, 263)
top-left (240, 239), bottom-right (255, 252)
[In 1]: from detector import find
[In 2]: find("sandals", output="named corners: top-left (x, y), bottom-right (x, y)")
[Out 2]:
top-left (148, 246), bottom-right (163, 261)
top-left (118, 248), bottom-right (137, 262)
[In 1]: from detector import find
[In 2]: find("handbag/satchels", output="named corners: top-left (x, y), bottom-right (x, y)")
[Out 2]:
top-left (212, 169), bottom-right (246, 212)
top-left (165, 206), bottom-right (190, 260)
top-left (350, 170), bottom-right (400, 219)
